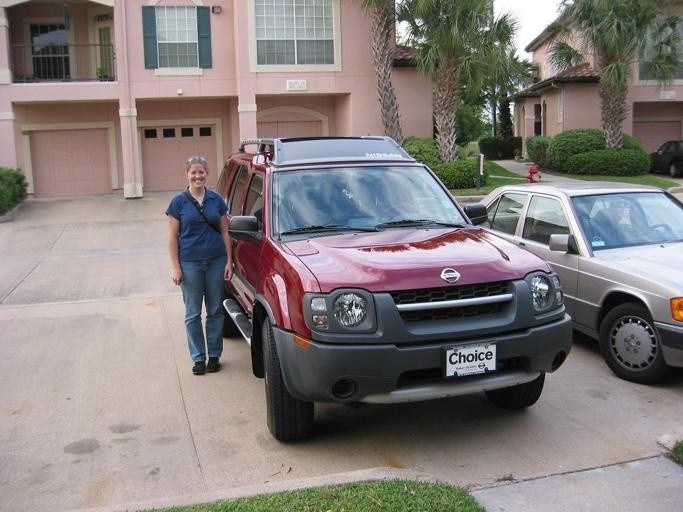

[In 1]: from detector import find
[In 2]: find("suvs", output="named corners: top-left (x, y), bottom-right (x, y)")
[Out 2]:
top-left (207, 131), bottom-right (576, 445)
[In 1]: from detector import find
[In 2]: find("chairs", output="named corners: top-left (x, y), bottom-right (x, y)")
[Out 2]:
top-left (532, 211), bottom-right (562, 238)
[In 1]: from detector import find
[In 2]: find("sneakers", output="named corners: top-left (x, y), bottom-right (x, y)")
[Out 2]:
top-left (206, 357), bottom-right (220, 371)
top-left (192, 361), bottom-right (205, 374)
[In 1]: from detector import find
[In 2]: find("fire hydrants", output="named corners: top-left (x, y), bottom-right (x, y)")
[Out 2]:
top-left (525, 161), bottom-right (542, 184)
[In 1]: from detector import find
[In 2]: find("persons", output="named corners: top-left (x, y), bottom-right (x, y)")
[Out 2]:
top-left (163, 156), bottom-right (234, 375)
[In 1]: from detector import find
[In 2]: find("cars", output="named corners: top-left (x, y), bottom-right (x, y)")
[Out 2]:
top-left (648, 139), bottom-right (682, 178)
top-left (474, 181), bottom-right (682, 384)
